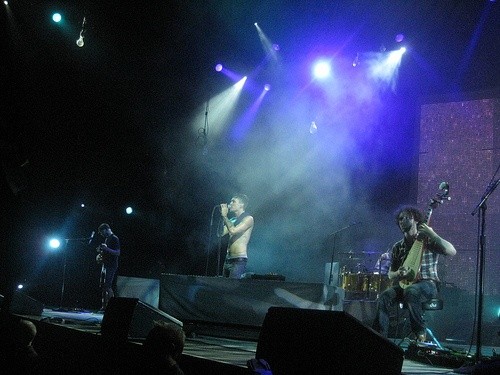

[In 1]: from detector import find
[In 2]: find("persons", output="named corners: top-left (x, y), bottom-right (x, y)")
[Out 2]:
top-left (15, 319), bottom-right (39, 358)
top-left (217, 193), bottom-right (254, 278)
top-left (143, 324), bottom-right (185, 374)
top-left (96, 223), bottom-right (120, 314)
top-left (371, 206), bottom-right (457, 343)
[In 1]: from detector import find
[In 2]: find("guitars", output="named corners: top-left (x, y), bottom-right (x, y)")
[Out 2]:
top-left (341, 271), bottom-right (389, 295)
top-left (398, 180), bottom-right (452, 288)
top-left (96, 231), bottom-right (105, 266)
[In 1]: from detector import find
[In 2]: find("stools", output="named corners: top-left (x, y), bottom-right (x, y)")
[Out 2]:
top-left (388, 298), bottom-right (443, 350)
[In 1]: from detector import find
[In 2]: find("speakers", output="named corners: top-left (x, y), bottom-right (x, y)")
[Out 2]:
top-left (39, 281), bottom-right (79, 306)
top-left (256, 307), bottom-right (404, 375)
top-left (0, 293), bottom-right (43, 316)
top-left (100, 296), bottom-right (183, 341)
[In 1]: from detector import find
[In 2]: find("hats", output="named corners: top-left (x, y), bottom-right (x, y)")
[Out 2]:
top-left (97, 223), bottom-right (109, 234)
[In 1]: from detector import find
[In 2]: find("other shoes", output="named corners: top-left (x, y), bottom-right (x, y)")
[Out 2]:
top-left (414, 331), bottom-right (427, 343)
top-left (98, 307), bottom-right (106, 312)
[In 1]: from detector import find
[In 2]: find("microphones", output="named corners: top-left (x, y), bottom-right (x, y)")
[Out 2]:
top-left (218, 204), bottom-right (231, 208)
top-left (89, 231), bottom-right (95, 243)
top-left (349, 221), bottom-right (360, 225)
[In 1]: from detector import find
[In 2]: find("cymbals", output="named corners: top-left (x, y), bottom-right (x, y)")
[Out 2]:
top-left (338, 248), bottom-right (383, 254)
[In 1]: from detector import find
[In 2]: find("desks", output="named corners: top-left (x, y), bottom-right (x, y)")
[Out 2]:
top-left (159, 272), bottom-right (347, 343)
top-left (116, 275), bottom-right (160, 309)
